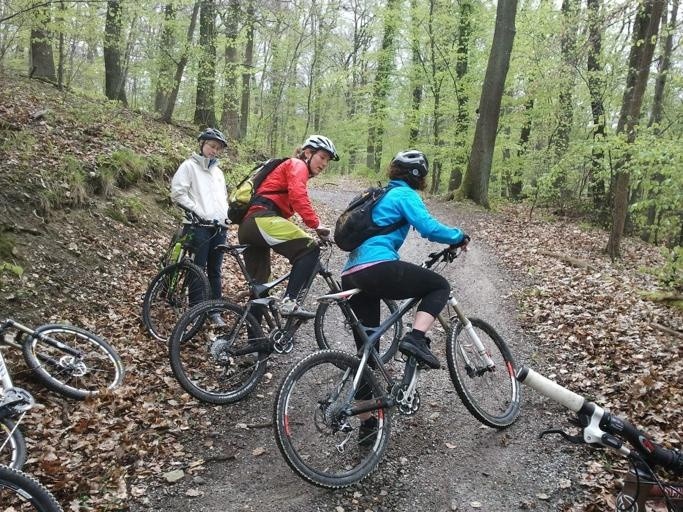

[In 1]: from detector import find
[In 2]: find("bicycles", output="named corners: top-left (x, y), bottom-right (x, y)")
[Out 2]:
top-left (514, 366), bottom-right (683, 511)
top-left (0, 302), bottom-right (124, 470)
top-left (0, 462), bottom-right (65, 512)
top-left (143, 201), bottom-right (231, 342)
top-left (273, 238), bottom-right (521, 489)
top-left (170, 231), bottom-right (402, 403)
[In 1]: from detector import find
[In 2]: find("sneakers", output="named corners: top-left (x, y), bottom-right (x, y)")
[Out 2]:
top-left (398, 330), bottom-right (441, 371)
top-left (357, 425), bottom-right (379, 446)
top-left (243, 351), bottom-right (272, 369)
top-left (209, 312), bottom-right (226, 327)
top-left (279, 297), bottom-right (317, 320)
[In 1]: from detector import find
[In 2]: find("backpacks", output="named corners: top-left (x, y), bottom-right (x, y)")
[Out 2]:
top-left (335, 181), bottom-right (409, 253)
top-left (227, 157), bottom-right (289, 223)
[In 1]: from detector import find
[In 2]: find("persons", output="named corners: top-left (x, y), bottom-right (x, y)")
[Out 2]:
top-left (169, 127), bottom-right (229, 331)
top-left (340, 149), bottom-right (470, 448)
top-left (238, 134), bottom-right (341, 361)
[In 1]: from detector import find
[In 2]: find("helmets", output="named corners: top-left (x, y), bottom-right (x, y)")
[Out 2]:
top-left (302, 134), bottom-right (340, 162)
top-left (196, 127), bottom-right (229, 148)
top-left (388, 149), bottom-right (430, 178)
